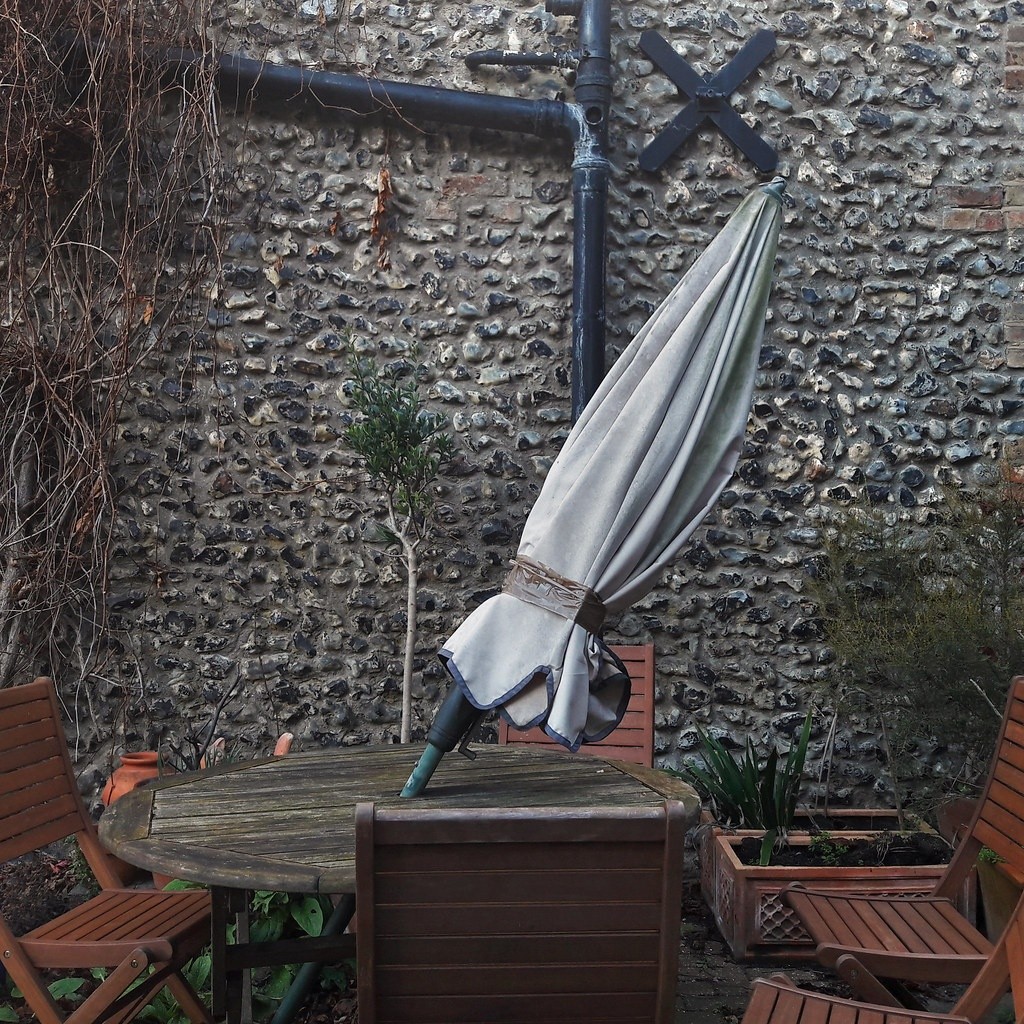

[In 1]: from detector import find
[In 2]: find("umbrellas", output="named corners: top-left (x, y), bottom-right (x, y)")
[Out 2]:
top-left (256, 172), bottom-right (795, 1023)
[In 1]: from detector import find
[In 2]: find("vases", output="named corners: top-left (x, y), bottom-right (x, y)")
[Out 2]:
top-left (711, 708), bottom-right (979, 963)
top-left (658, 708), bottom-right (939, 912)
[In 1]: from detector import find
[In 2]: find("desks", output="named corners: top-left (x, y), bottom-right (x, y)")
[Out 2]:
top-left (98, 742), bottom-right (700, 1022)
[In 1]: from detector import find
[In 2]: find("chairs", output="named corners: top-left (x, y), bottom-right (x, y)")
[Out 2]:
top-left (498, 643), bottom-right (656, 765)
top-left (0, 676), bottom-right (255, 1024)
top-left (738, 889), bottom-right (1024, 1024)
top-left (778, 674), bottom-right (1024, 1008)
top-left (350, 799), bottom-right (683, 1024)
